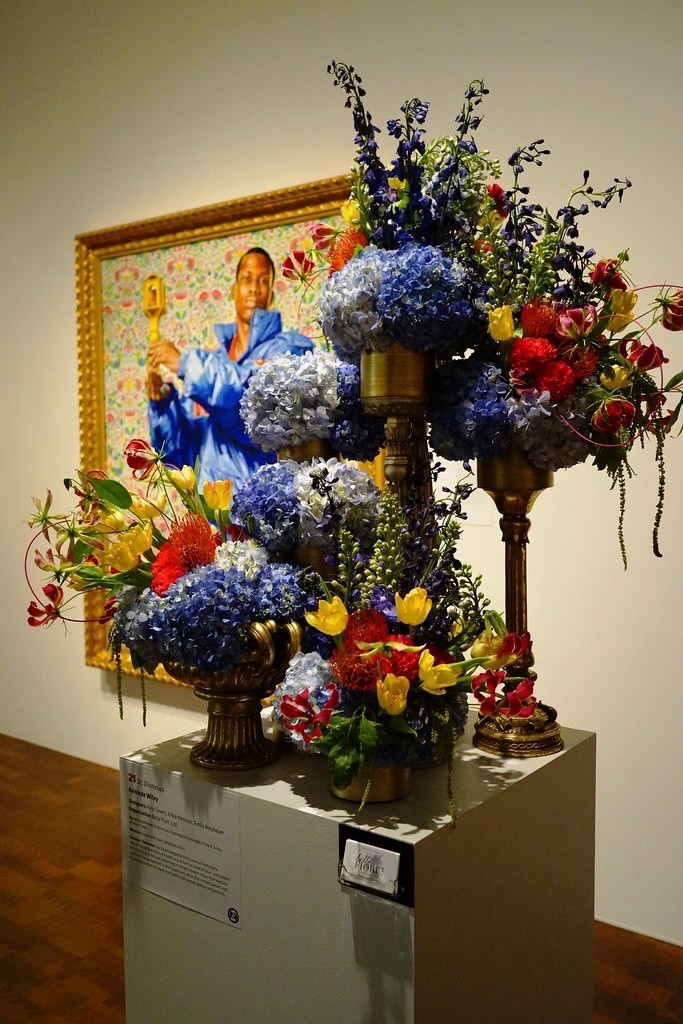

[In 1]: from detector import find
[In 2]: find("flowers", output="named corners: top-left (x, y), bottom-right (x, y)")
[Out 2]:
top-left (26, 60), bottom-right (673, 777)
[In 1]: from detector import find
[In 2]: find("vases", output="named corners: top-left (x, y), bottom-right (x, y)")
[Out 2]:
top-left (181, 607), bottom-right (300, 771)
top-left (316, 682), bottom-right (415, 803)
top-left (343, 329), bottom-right (439, 548)
top-left (474, 431), bottom-right (564, 754)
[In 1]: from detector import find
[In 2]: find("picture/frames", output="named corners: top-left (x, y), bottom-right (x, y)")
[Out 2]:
top-left (70, 172), bottom-right (385, 703)
